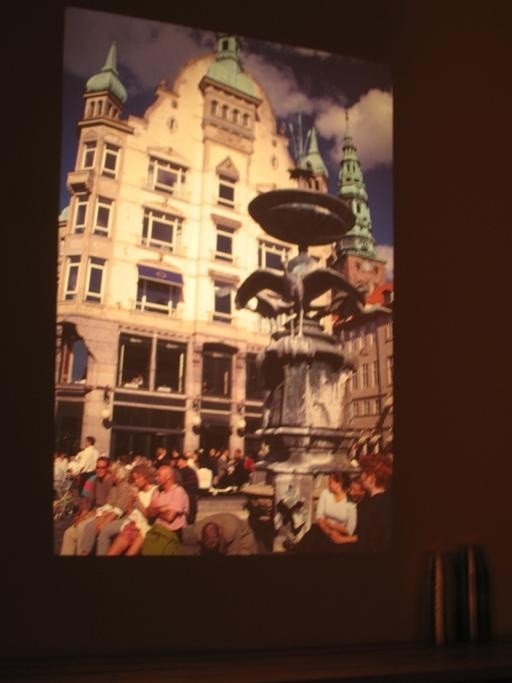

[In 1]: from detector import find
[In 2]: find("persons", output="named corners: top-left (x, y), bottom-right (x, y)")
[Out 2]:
top-left (53, 371), bottom-right (397, 557)
